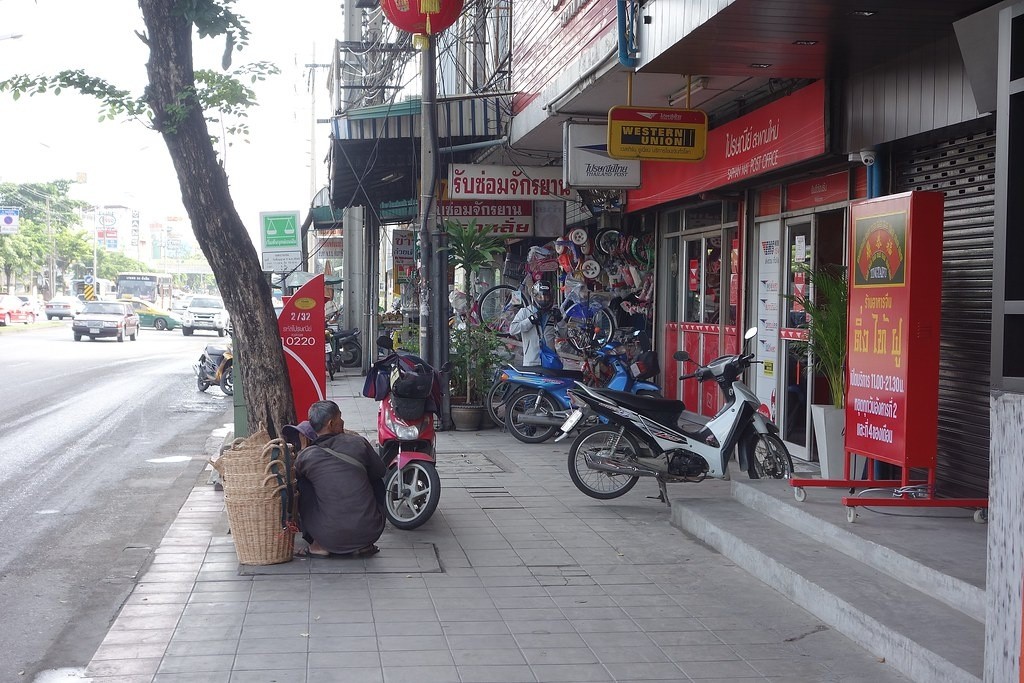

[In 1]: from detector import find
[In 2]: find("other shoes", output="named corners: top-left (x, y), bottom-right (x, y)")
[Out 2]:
top-left (551, 432), bottom-right (564, 437)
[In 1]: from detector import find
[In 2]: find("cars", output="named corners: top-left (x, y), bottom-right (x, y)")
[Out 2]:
top-left (43, 297), bottom-right (182, 342)
top-left (0, 297), bottom-right (38, 326)
top-left (171, 293), bottom-right (231, 338)
top-left (271, 289), bottom-right (285, 313)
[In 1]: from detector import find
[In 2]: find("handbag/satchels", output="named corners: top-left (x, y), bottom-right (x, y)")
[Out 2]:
top-left (629, 351), bottom-right (660, 378)
top-left (539, 346), bottom-right (563, 370)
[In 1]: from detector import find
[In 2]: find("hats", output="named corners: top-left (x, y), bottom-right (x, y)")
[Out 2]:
top-left (281, 421), bottom-right (317, 450)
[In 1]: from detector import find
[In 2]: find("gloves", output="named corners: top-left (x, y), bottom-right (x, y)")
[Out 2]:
top-left (553, 310), bottom-right (563, 321)
top-left (528, 312), bottom-right (543, 324)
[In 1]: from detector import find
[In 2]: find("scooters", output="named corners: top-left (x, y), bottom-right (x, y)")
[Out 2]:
top-left (365, 336), bottom-right (453, 529)
top-left (487, 329), bottom-right (795, 499)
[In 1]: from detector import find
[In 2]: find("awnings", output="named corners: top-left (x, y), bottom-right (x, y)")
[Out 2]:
top-left (333, 92), bottom-right (511, 211)
top-left (311, 198), bottom-right (426, 228)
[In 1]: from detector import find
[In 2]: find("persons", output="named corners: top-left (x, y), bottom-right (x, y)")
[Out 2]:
top-left (509, 279), bottom-right (568, 438)
top-left (293, 401), bottom-right (386, 558)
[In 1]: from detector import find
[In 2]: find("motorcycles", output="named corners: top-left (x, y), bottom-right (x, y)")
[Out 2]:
top-left (325, 323), bottom-right (362, 380)
top-left (193, 346), bottom-right (236, 396)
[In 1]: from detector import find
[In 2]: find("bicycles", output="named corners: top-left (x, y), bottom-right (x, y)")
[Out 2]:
top-left (482, 274), bottom-right (614, 353)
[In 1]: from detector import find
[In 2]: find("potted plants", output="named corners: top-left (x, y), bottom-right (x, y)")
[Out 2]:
top-left (434, 217), bottom-right (515, 431)
top-left (778, 259), bottom-right (867, 483)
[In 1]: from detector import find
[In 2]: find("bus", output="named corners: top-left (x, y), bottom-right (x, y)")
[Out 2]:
top-left (116, 272), bottom-right (176, 305)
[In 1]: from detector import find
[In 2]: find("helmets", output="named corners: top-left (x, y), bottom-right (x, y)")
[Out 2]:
top-left (532, 280), bottom-right (555, 310)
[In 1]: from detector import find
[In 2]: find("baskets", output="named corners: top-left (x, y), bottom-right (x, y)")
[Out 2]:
top-left (503, 253), bottom-right (526, 280)
top-left (207, 420), bottom-right (299, 566)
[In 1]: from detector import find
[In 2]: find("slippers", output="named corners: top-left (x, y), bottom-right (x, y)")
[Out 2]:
top-left (353, 545), bottom-right (380, 559)
top-left (295, 546), bottom-right (330, 559)
